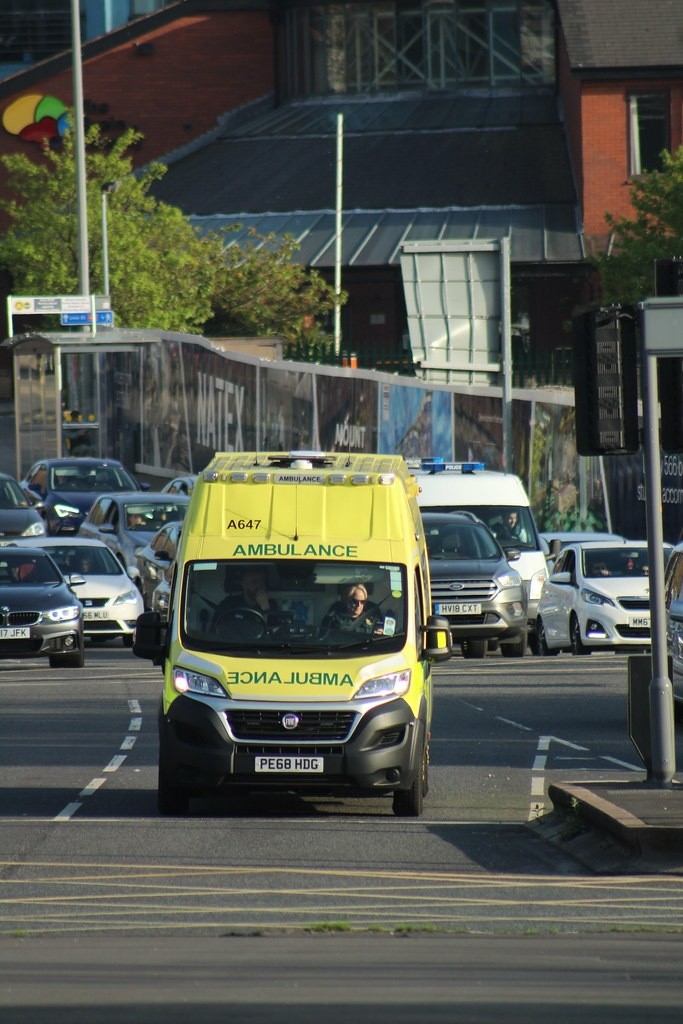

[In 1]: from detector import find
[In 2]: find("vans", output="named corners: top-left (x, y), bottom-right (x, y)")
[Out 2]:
top-left (129, 449), bottom-right (458, 817)
top-left (400, 455), bottom-right (564, 656)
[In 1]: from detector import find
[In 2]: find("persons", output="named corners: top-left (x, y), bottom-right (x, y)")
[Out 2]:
top-left (320, 584), bottom-right (383, 638)
top-left (54, 476), bottom-right (76, 489)
top-left (590, 560), bottom-right (609, 578)
top-left (127, 513), bottom-right (146, 531)
top-left (79, 556), bottom-right (91, 573)
top-left (491, 509), bottom-right (528, 544)
top-left (6, 559), bottom-right (38, 583)
top-left (639, 564), bottom-right (649, 576)
top-left (209, 570), bottom-right (279, 640)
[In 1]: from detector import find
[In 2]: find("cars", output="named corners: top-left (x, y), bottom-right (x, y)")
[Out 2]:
top-left (3, 535), bottom-right (146, 650)
top-left (161, 474), bottom-right (200, 496)
top-left (126, 519), bottom-right (186, 650)
top-left (15, 455), bottom-right (151, 538)
top-left (537, 531), bottom-right (624, 577)
top-left (534, 539), bottom-right (673, 654)
top-left (74, 491), bottom-right (193, 578)
top-left (1, 472), bottom-right (45, 546)
top-left (0, 543), bottom-right (87, 669)
top-left (662, 538), bottom-right (683, 707)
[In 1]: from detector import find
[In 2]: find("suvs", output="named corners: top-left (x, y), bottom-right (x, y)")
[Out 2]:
top-left (417, 508), bottom-right (530, 658)
